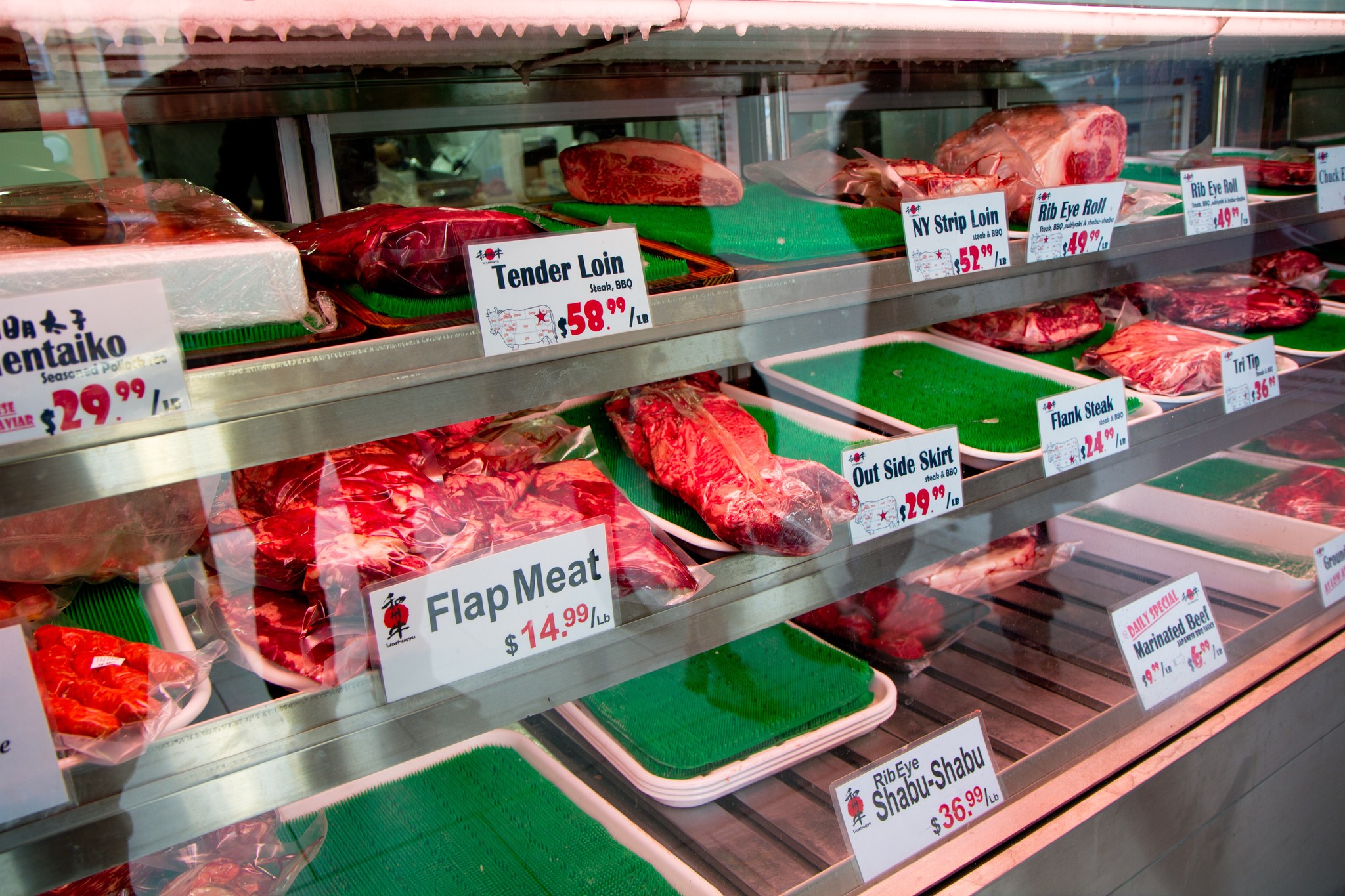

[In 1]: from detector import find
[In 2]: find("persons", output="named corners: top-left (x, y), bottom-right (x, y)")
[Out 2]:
top-left (831, 46), bottom-right (1113, 700)
top-left (125, 26), bottom-right (489, 780)
top-left (507, 52), bottom-right (873, 718)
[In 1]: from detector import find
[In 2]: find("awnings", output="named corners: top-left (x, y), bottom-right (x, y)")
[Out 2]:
top-left (2, 0), bottom-right (1345, 109)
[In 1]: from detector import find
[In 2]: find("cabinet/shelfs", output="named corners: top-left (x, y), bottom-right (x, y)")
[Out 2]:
top-left (0, 1), bottom-right (1344, 896)
